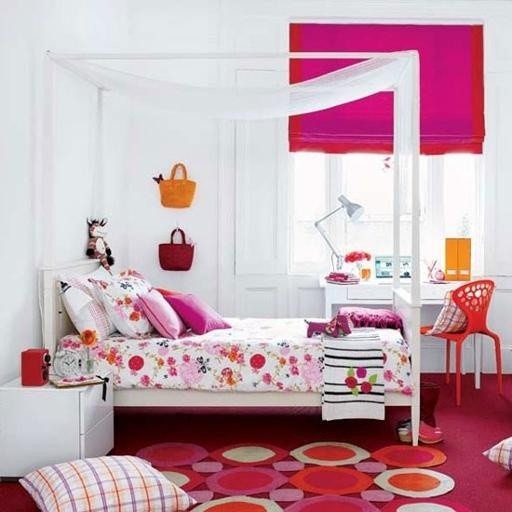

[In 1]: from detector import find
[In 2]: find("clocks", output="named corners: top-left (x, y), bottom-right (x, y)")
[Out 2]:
top-left (54, 349), bottom-right (82, 377)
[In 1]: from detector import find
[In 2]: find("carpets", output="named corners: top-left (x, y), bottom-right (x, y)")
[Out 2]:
top-left (0, 372), bottom-right (512, 512)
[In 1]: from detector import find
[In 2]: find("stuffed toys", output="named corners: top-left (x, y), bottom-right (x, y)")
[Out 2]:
top-left (86, 216), bottom-right (114, 275)
top-left (304, 310), bottom-right (352, 338)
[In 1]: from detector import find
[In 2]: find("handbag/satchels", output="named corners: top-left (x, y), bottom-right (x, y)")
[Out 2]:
top-left (160, 163), bottom-right (195, 207)
top-left (159, 229), bottom-right (194, 271)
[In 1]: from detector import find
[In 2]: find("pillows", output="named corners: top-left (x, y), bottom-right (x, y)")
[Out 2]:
top-left (57, 265), bottom-right (117, 340)
top-left (88, 267), bottom-right (153, 338)
top-left (19, 455), bottom-right (198, 512)
top-left (156, 288), bottom-right (191, 330)
top-left (339, 307), bottom-right (403, 326)
top-left (425, 290), bottom-right (479, 336)
top-left (164, 294), bottom-right (232, 335)
top-left (481, 436), bottom-right (512, 472)
top-left (136, 289), bottom-right (186, 339)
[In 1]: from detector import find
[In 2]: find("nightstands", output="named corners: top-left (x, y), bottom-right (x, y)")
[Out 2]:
top-left (1, 374), bottom-right (115, 477)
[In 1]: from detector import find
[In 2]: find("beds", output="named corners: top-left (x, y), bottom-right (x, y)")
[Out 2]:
top-left (38, 51), bottom-right (420, 446)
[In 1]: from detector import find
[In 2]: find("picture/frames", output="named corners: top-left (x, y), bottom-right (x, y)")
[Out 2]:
top-left (375, 256), bottom-right (412, 278)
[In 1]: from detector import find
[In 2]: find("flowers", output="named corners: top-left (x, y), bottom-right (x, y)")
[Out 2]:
top-left (78, 330), bottom-right (99, 374)
top-left (344, 251), bottom-right (371, 263)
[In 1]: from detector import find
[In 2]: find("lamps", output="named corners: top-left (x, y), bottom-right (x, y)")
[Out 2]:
top-left (314, 195), bottom-right (364, 271)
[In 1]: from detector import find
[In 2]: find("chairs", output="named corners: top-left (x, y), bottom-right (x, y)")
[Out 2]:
top-left (420, 280), bottom-right (503, 407)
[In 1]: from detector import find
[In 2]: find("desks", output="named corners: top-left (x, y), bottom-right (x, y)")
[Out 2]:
top-left (319, 273), bottom-right (482, 389)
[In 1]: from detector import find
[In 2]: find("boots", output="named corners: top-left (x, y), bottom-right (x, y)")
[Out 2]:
top-left (398, 381), bottom-right (441, 428)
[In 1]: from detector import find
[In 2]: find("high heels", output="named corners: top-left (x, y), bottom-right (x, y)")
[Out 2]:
top-left (398, 420), bottom-right (442, 432)
top-left (399, 431), bottom-right (443, 443)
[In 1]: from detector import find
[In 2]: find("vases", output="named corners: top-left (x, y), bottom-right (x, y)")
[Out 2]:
top-left (356, 262), bottom-right (362, 271)
